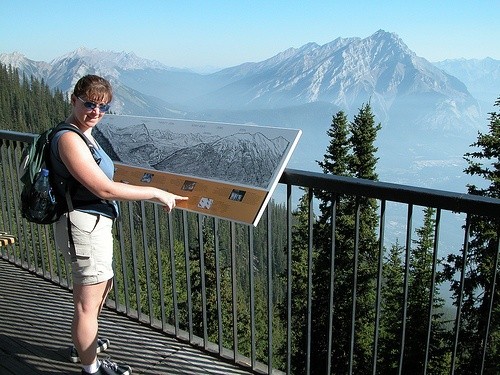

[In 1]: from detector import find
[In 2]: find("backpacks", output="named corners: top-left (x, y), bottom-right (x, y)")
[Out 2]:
top-left (18, 120), bottom-right (102, 224)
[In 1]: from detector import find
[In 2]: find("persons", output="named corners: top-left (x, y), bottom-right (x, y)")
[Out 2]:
top-left (43, 74), bottom-right (189, 374)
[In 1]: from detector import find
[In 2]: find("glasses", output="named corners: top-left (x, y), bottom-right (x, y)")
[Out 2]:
top-left (78, 96), bottom-right (111, 112)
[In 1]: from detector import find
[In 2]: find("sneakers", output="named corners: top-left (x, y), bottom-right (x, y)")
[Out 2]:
top-left (80, 354), bottom-right (132, 375)
top-left (69, 337), bottom-right (110, 363)
